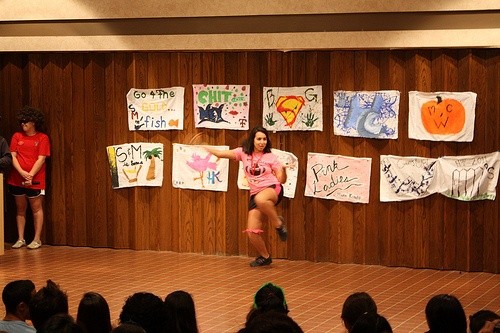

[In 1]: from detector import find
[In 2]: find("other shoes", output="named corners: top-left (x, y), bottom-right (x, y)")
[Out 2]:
top-left (12, 240), bottom-right (26, 248)
top-left (27, 240), bottom-right (42, 249)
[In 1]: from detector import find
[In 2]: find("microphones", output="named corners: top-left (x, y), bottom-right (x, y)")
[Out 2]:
top-left (21, 181), bottom-right (40, 185)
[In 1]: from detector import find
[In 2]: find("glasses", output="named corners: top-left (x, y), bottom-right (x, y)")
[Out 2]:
top-left (17, 118), bottom-right (32, 125)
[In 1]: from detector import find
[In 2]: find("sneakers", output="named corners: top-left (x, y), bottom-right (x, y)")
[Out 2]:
top-left (276, 216), bottom-right (288, 241)
top-left (250, 256), bottom-right (271, 267)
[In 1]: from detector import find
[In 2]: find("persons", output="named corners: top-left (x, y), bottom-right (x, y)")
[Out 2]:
top-left (0, 136), bottom-right (12, 184)
top-left (0, 277), bottom-right (199, 333)
top-left (7, 107), bottom-right (50, 249)
top-left (203, 127), bottom-right (288, 267)
top-left (469, 309), bottom-right (500, 333)
top-left (425, 294), bottom-right (467, 333)
top-left (342, 292), bottom-right (393, 333)
top-left (237, 282), bottom-right (304, 333)
